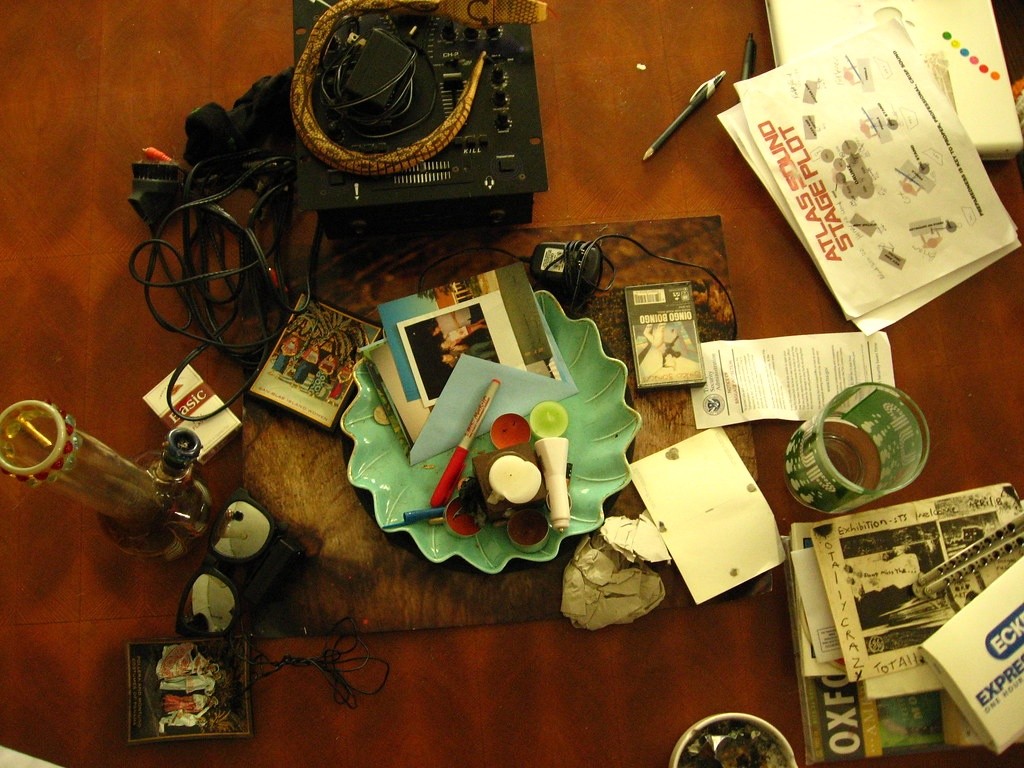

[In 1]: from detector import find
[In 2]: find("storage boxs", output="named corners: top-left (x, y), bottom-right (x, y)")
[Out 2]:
top-left (625, 280), bottom-right (706, 390)
top-left (143, 362), bottom-right (243, 465)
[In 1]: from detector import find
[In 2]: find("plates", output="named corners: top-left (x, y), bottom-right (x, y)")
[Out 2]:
top-left (340, 291), bottom-right (641, 575)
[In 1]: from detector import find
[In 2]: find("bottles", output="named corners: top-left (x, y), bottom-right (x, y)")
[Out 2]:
top-left (0, 400), bottom-right (214, 561)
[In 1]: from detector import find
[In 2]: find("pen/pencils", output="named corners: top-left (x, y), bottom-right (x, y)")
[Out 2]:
top-left (642, 70), bottom-right (727, 160)
top-left (429, 379), bottom-right (501, 508)
top-left (737, 31), bottom-right (756, 103)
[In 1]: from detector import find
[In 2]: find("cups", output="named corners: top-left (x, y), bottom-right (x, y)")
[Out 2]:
top-left (783, 383), bottom-right (930, 515)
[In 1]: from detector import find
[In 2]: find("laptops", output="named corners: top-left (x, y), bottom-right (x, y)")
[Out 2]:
top-left (763, 0), bottom-right (1022, 162)
